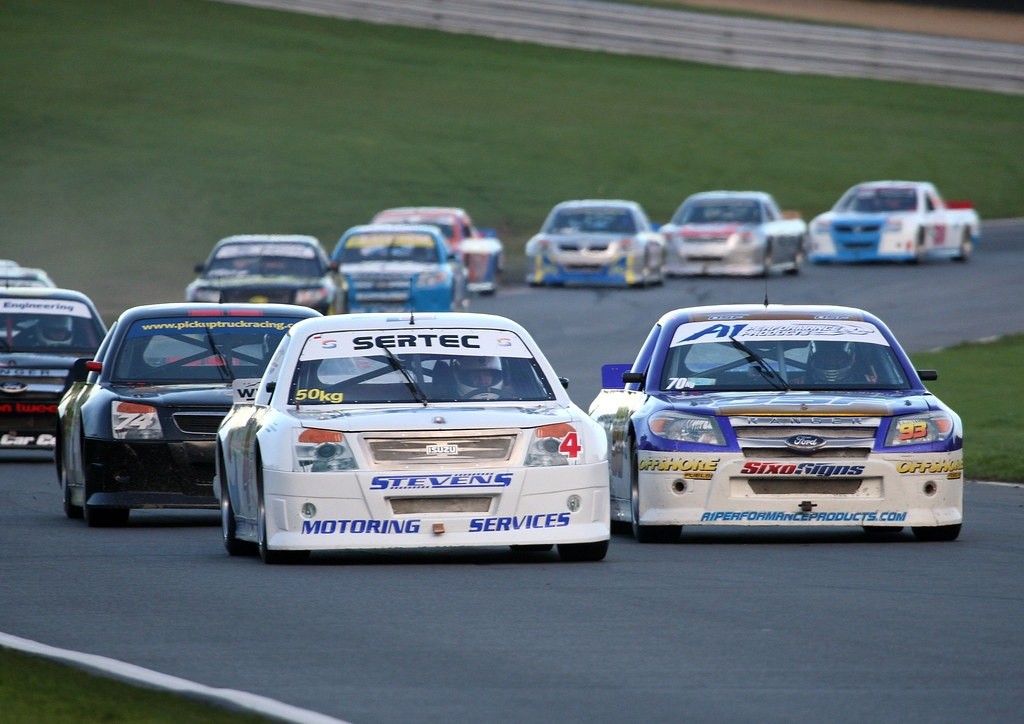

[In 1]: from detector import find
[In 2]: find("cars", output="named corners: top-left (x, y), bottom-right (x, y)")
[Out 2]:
top-left (659, 189), bottom-right (811, 276)
top-left (0, 257), bottom-right (109, 448)
top-left (588, 271), bottom-right (963, 541)
top-left (56, 301), bottom-right (323, 526)
top-left (811, 180), bottom-right (981, 268)
top-left (330, 206), bottom-right (504, 313)
top-left (212, 277), bottom-right (610, 564)
top-left (185, 234), bottom-right (343, 317)
top-left (524, 200), bottom-right (671, 288)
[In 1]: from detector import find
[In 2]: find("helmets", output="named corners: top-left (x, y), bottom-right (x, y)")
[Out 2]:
top-left (35, 315), bottom-right (73, 349)
top-left (451, 356), bottom-right (502, 401)
top-left (807, 340), bottom-right (856, 380)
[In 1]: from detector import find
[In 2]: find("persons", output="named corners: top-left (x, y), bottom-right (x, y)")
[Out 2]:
top-left (450, 356), bottom-right (503, 400)
top-left (794, 341), bottom-right (856, 384)
top-left (34, 315), bottom-right (74, 349)
top-left (262, 333), bottom-right (286, 361)
